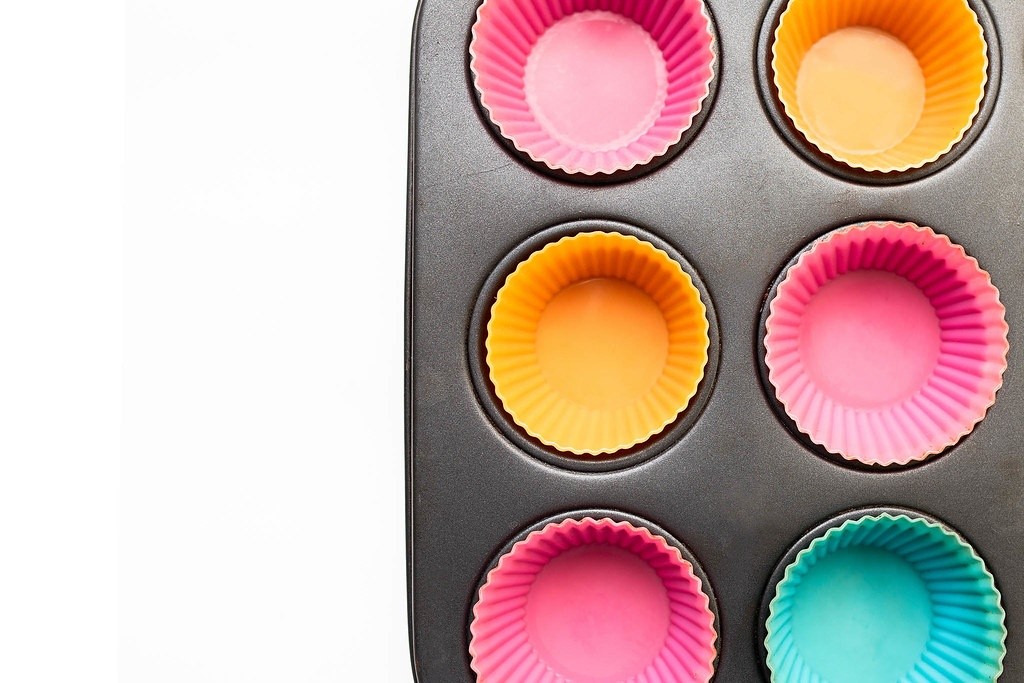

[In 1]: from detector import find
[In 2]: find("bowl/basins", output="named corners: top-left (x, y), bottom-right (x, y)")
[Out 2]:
top-left (763, 220), bottom-right (1009, 467)
top-left (772, 0), bottom-right (989, 173)
top-left (764, 510), bottom-right (1006, 683)
top-left (469, 0), bottom-right (715, 176)
top-left (484, 232), bottom-right (710, 455)
top-left (468, 517), bottom-right (717, 683)
